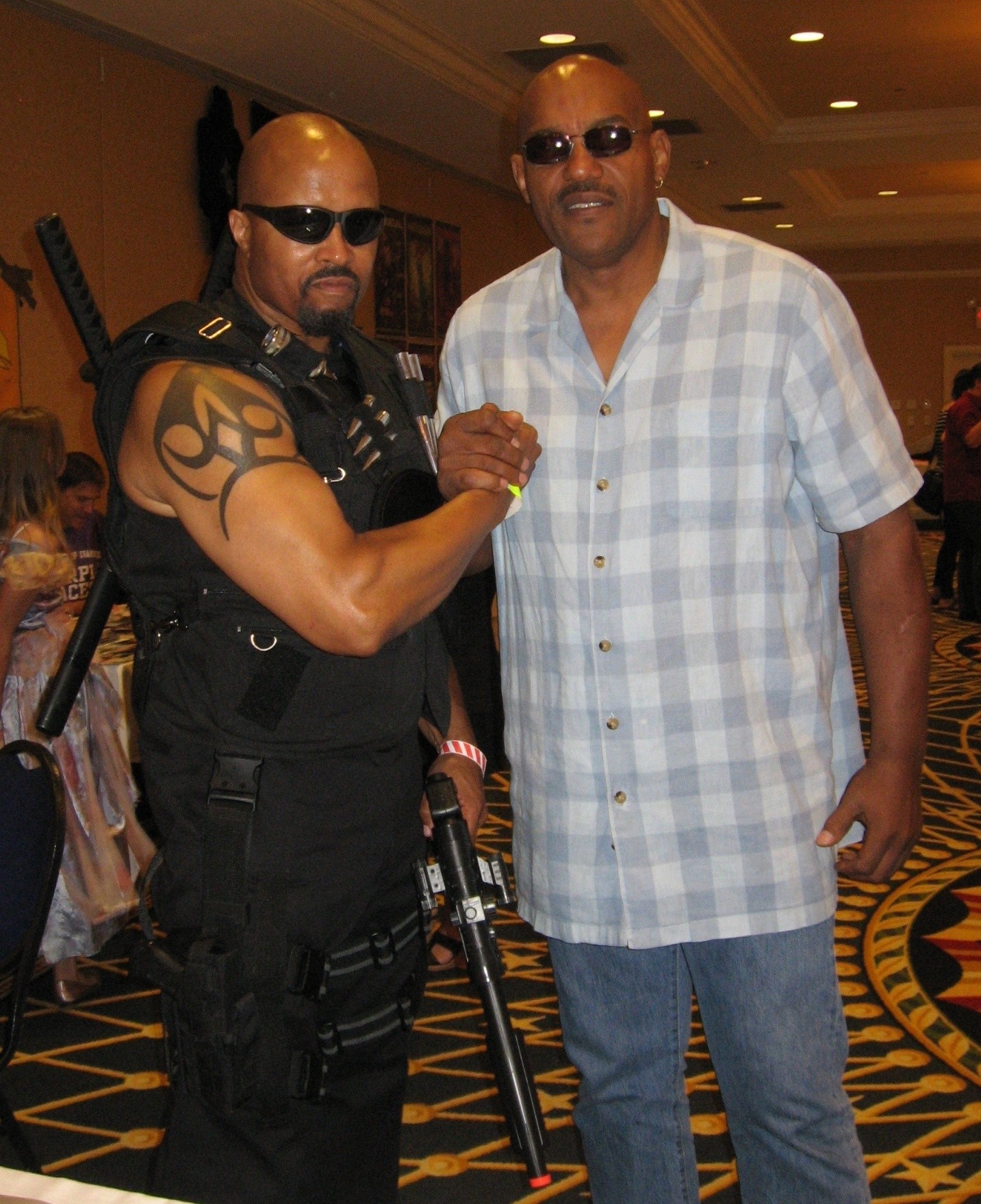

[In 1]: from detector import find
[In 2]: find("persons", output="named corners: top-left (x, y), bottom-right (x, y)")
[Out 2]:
top-left (57, 452), bottom-right (126, 617)
top-left (942, 361), bottom-right (981, 624)
top-left (91, 111), bottom-right (543, 1204)
top-left (1, 406), bottom-right (159, 1003)
top-left (434, 53), bottom-right (932, 1203)
top-left (930, 369), bottom-right (971, 610)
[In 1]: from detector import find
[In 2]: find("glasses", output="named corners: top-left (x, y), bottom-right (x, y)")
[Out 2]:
top-left (240, 203), bottom-right (386, 246)
top-left (517, 124), bottom-right (653, 165)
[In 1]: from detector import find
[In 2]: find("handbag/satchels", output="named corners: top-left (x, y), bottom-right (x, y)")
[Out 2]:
top-left (913, 469), bottom-right (946, 516)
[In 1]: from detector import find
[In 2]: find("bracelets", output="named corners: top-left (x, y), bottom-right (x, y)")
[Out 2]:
top-left (438, 740), bottom-right (487, 779)
top-left (504, 484), bottom-right (522, 520)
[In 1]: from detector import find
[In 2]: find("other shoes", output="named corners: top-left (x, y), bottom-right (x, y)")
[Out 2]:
top-left (56, 968), bottom-right (101, 1002)
top-left (930, 595), bottom-right (960, 609)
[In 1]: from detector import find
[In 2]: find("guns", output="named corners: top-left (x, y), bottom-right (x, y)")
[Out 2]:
top-left (409, 770), bottom-right (557, 1191)
